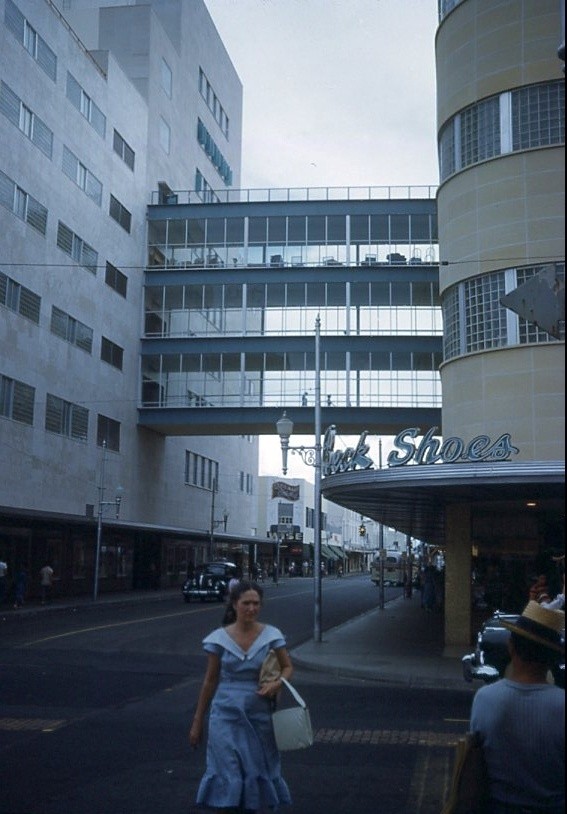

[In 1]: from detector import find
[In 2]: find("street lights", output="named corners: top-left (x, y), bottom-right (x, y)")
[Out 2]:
top-left (92, 430), bottom-right (124, 602)
top-left (209, 477), bottom-right (230, 560)
top-left (277, 311), bottom-right (323, 642)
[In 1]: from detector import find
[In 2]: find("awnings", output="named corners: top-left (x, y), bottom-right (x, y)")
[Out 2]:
top-left (320, 545), bottom-right (339, 561)
top-left (330, 546), bottom-right (348, 559)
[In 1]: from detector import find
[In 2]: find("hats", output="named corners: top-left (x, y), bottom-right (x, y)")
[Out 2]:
top-left (502, 600), bottom-right (565, 653)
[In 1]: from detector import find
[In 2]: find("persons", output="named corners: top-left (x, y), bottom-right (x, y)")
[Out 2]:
top-left (12, 562), bottom-right (30, 610)
top-left (421, 565), bottom-right (444, 613)
top-left (288, 564), bottom-right (294, 578)
top-left (229, 572), bottom-right (240, 593)
top-left (39, 560), bottom-right (54, 605)
top-left (469, 601), bottom-right (565, 814)
top-left (188, 582), bottom-right (293, 814)
top-left (529, 572), bottom-right (551, 604)
top-left (302, 560), bottom-right (308, 577)
top-left (308, 560), bottom-right (314, 577)
top-left (337, 566), bottom-right (343, 580)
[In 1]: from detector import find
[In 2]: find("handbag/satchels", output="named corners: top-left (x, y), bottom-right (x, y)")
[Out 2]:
top-left (271, 678), bottom-right (313, 752)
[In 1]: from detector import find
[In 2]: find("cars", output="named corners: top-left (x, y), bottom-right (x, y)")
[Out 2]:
top-left (461, 608), bottom-right (567, 686)
top-left (182, 561), bottom-right (242, 601)
top-left (370, 549), bottom-right (425, 589)
